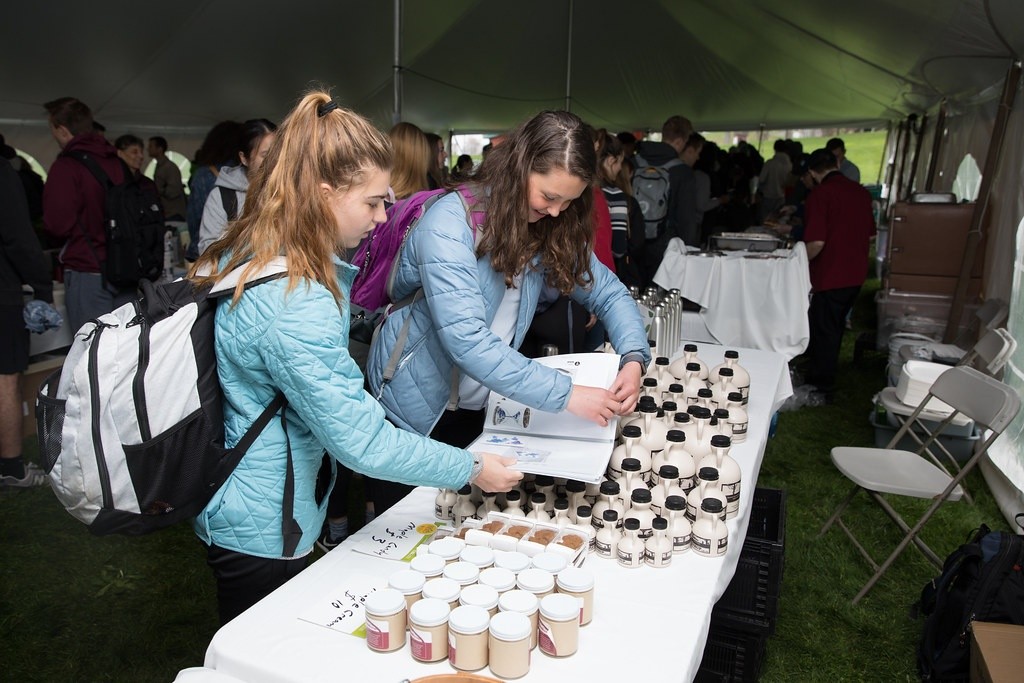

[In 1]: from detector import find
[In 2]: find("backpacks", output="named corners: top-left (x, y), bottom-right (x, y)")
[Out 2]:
top-left (57, 149), bottom-right (165, 297)
top-left (629, 154), bottom-right (686, 243)
top-left (908, 513), bottom-right (1024, 683)
top-left (349, 182), bottom-right (489, 383)
top-left (35, 254), bottom-right (342, 557)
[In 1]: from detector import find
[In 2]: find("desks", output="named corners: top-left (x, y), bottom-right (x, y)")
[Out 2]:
top-left (179, 340), bottom-right (795, 683)
top-left (23, 268), bottom-right (192, 356)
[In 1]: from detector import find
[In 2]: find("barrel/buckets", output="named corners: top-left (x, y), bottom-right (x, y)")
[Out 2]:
top-left (885, 333), bottom-right (935, 390)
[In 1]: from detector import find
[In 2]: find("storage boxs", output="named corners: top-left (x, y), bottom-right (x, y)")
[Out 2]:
top-left (869, 393), bottom-right (980, 464)
top-left (896, 359), bottom-right (963, 412)
top-left (873, 288), bottom-right (954, 348)
top-left (692, 488), bottom-right (786, 682)
top-left (969, 620), bottom-right (1024, 683)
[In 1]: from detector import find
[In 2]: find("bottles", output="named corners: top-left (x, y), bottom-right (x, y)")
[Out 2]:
top-left (875, 391), bottom-right (887, 426)
top-left (366, 284), bottom-right (751, 679)
top-left (108, 219), bottom-right (123, 241)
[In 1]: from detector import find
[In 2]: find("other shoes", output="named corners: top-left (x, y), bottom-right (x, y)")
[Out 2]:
top-left (0, 460), bottom-right (46, 489)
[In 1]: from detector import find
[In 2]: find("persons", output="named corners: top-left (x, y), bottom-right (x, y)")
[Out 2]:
top-left (517, 117), bottom-right (878, 385)
top-left (188, 119), bottom-right (246, 262)
top-left (316, 454), bottom-right (382, 555)
top-left (147, 135), bottom-right (185, 223)
top-left (198, 119), bottom-right (278, 256)
top-left (92, 121), bottom-right (107, 136)
top-left (424, 132), bottom-right (448, 190)
top-left (363, 108), bottom-right (652, 517)
top-left (115, 135), bottom-right (166, 282)
top-left (39, 97), bottom-right (138, 343)
top-left (0, 134), bottom-right (56, 487)
top-left (184, 79), bottom-right (524, 628)
top-left (452, 154), bottom-right (474, 181)
top-left (387, 121), bottom-right (431, 207)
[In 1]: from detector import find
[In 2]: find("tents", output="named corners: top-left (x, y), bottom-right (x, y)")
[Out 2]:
top-left (0, 0), bottom-right (1024, 379)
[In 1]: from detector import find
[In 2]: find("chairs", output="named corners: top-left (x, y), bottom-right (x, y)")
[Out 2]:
top-left (899, 295), bottom-right (1008, 367)
top-left (880, 328), bottom-right (1016, 502)
top-left (815, 365), bottom-right (1021, 607)
